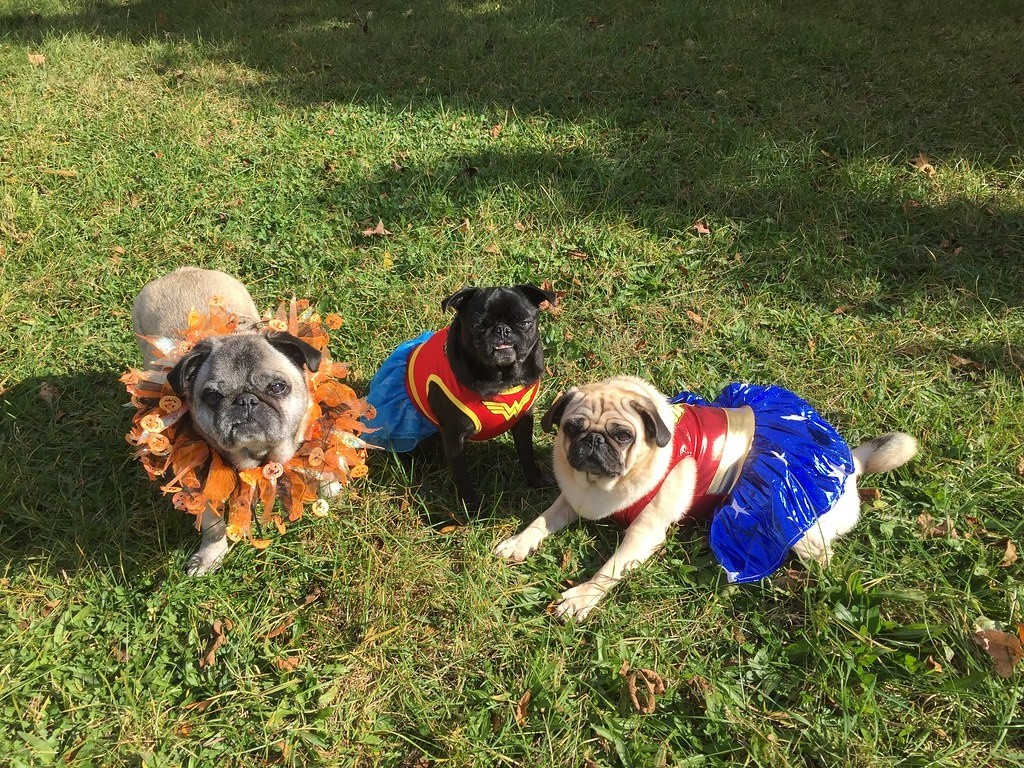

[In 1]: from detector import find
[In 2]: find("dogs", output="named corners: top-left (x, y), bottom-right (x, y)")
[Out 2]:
top-left (131, 264), bottom-right (920, 623)
